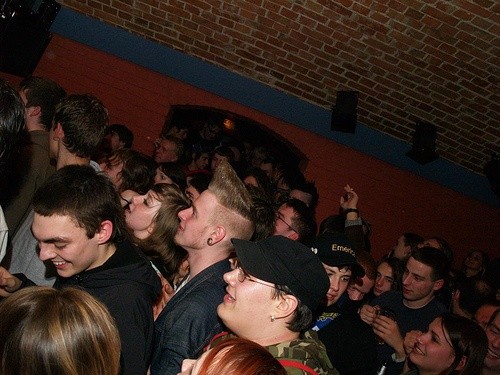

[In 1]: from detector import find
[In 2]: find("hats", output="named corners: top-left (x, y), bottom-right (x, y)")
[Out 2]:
top-left (309, 233), bottom-right (365, 277)
top-left (231, 235), bottom-right (330, 316)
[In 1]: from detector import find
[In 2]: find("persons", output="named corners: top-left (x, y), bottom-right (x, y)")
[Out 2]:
top-left (0, 74), bottom-right (499, 375)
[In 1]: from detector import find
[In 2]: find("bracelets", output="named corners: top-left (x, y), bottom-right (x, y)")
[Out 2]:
top-left (345, 208), bottom-right (359, 215)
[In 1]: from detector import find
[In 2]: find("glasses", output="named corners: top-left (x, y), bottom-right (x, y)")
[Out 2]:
top-left (231, 257), bottom-right (294, 295)
top-left (275, 214), bottom-right (299, 234)
